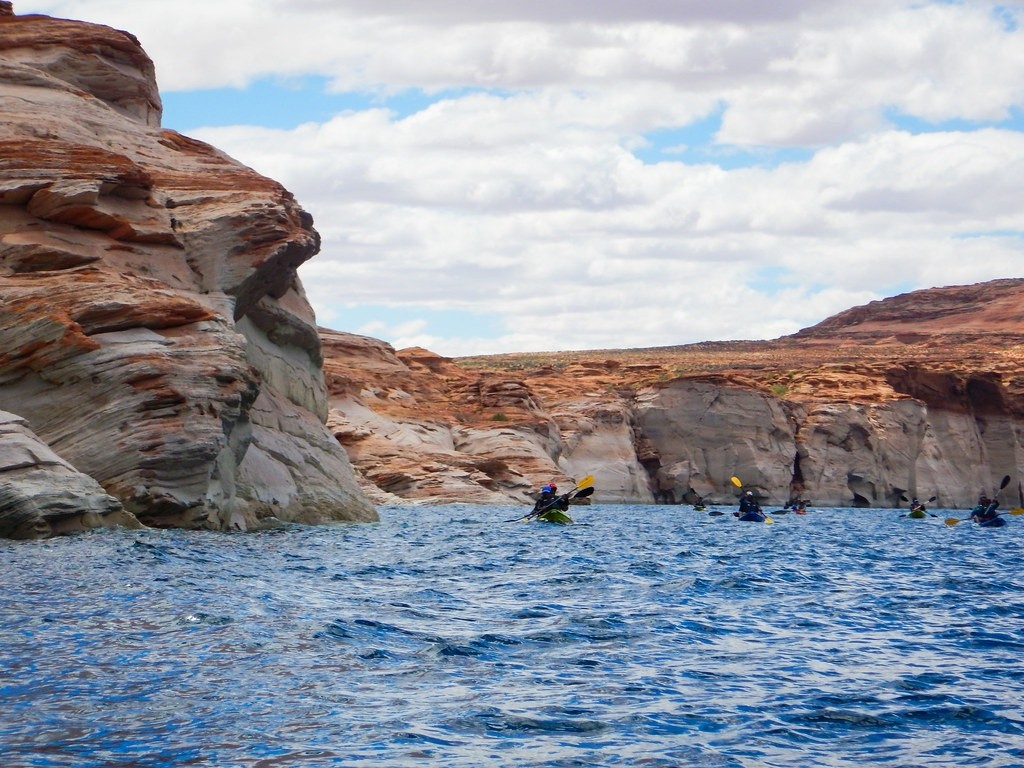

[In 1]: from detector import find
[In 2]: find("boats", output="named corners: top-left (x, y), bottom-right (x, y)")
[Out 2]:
top-left (693, 506), bottom-right (705, 512)
top-left (979, 516), bottom-right (1008, 527)
top-left (908, 510), bottom-right (930, 517)
top-left (770, 508), bottom-right (807, 514)
top-left (537, 509), bottom-right (576, 524)
top-left (737, 511), bottom-right (766, 522)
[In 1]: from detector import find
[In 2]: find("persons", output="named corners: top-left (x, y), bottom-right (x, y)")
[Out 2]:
top-left (694, 497), bottom-right (705, 507)
top-left (967, 494), bottom-right (999, 523)
top-left (528, 484), bottom-right (569, 519)
top-left (910, 498), bottom-right (925, 512)
top-left (784, 500), bottom-right (806, 511)
top-left (739, 492), bottom-right (762, 515)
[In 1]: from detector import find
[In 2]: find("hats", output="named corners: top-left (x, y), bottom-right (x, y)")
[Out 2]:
top-left (747, 491), bottom-right (752, 496)
top-left (549, 483), bottom-right (557, 490)
top-left (541, 486), bottom-right (551, 494)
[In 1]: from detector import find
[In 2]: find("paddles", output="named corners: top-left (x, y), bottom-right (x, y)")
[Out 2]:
top-left (900, 496), bottom-right (937, 519)
top-left (506, 486), bottom-right (594, 522)
top-left (731, 476), bottom-right (772, 521)
top-left (945, 509), bottom-right (1024, 526)
top-left (982, 475), bottom-right (1010, 520)
top-left (523, 475), bottom-right (592, 522)
top-left (708, 509), bottom-right (787, 517)
top-left (900, 493), bottom-right (938, 518)
